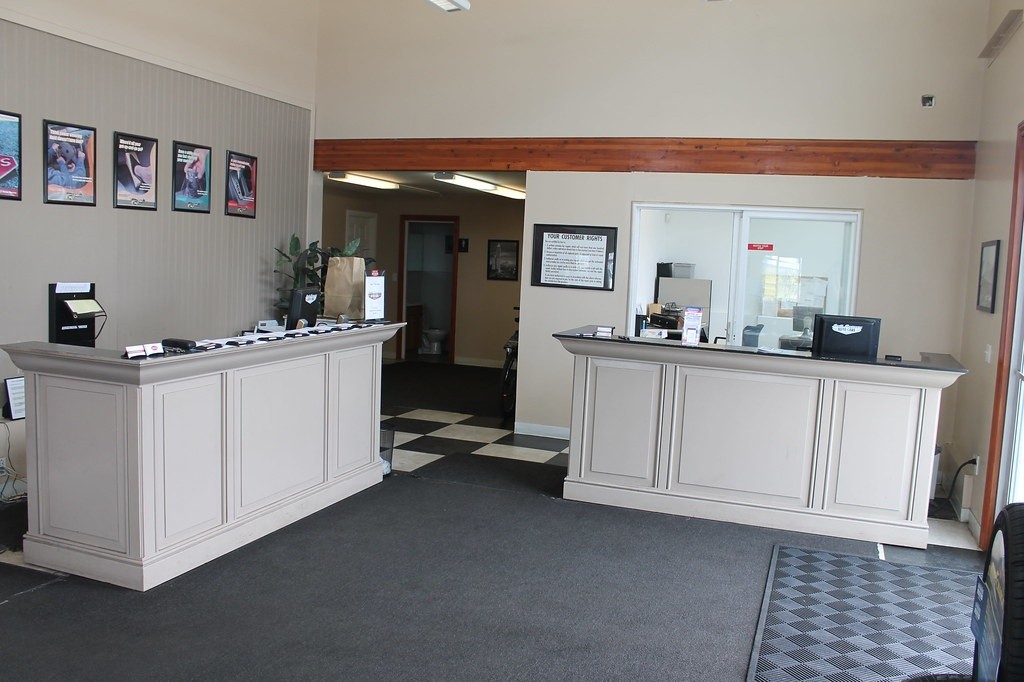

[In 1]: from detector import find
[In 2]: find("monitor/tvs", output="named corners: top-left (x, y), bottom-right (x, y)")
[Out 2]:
top-left (285, 287), bottom-right (320, 337)
top-left (811, 315), bottom-right (881, 365)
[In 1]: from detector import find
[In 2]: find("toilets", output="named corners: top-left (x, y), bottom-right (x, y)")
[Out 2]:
top-left (417, 328), bottom-right (449, 354)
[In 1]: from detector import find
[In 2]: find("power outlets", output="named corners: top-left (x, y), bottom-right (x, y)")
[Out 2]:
top-left (0, 457), bottom-right (7, 476)
top-left (970, 453), bottom-right (979, 476)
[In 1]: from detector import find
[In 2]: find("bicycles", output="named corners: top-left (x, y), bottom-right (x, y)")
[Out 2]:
top-left (498, 306), bottom-right (520, 412)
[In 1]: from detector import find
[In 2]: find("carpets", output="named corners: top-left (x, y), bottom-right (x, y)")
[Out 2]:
top-left (0, 474), bottom-right (880, 682)
top-left (745, 544), bottom-right (984, 682)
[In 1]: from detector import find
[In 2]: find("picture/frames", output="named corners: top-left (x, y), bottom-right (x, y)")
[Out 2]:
top-left (976, 240), bottom-right (1000, 313)
top-left (0, 110), bottom-right (22, 201)
top-left (113, 131), bottom-right (158, 211)
top-left (487, 239), bottom-right (519, 281)
top-left (43, 119), bottom-right (97, 207)
top-left (225, 150), bottom-right (257, 219)
top-left (171, 140), bottom-right (212, 214)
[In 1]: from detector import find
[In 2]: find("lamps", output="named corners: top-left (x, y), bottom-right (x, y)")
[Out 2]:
top-left (327, 173), bottom-right (399, 189)
top-left (433, 172), bottom-right (526, 199)
top-left (424, 0), bottom-right (471, 14)
